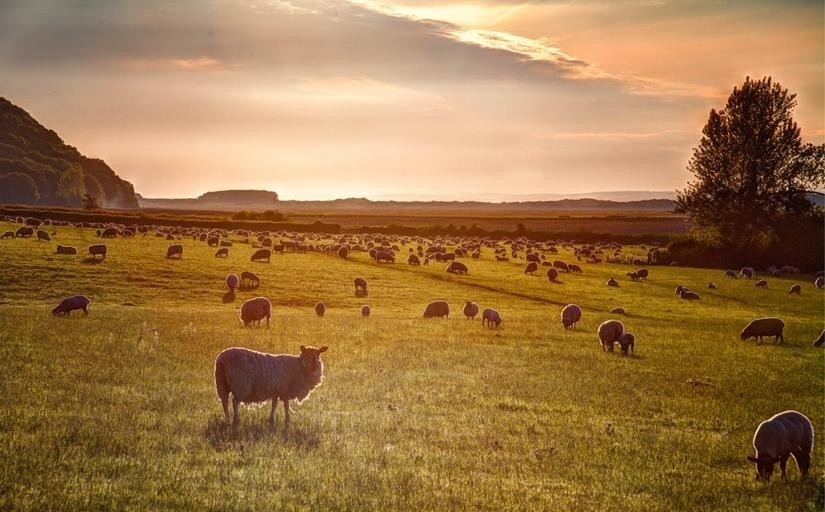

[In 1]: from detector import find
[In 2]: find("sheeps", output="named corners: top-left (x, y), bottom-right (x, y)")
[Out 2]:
top-left (707, 282), bottom-right (716, 288)
top-left (240, 296), bottom-right (271, 328)
top-left (596, 308), bottom-right (635, 355)
top-left (51, 295), bottom-right (92, 317)
top-left (0, 216), bottom-right (666, 291)
top-left (560, 304), bottom-right (583, 330)
top-left (746, 409), bottom-right (815, 484)
top-left (740, 316), bottom-right (786, 344)
top-left (362, 304), bottom-right (370, 317)
top-left (724, 263), bottom-right (824, 296)
top-left (482, 308), bottom-right (503, 328)
top-left (315, 302), bottom-right (326, 316)
top-left (214, 343), bottom-right (329, 425)
top-left (423, 301), bottom-right (450, 320)
top-left (462, 299), bottom-right (479, 320)
top-left (674, 284), bottom-right (700, 299)
top-left (813, 329), bottom-right (825, 348)
